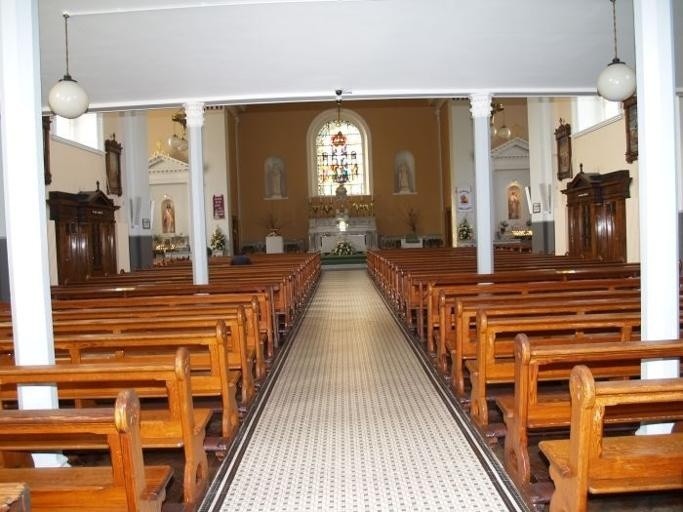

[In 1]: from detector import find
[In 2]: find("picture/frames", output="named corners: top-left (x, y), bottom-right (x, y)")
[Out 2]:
top-left (41, 116), bottom-right (52, 185)
top-left (104, 133), bottom-right (122, 197)
top-left (623, 96), bottom-right (638, 163)
top-left (553, 117), bottom-right (572, 181)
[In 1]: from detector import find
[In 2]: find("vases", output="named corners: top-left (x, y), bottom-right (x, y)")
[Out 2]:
top-left (211, 249), bottom-right (223, 257)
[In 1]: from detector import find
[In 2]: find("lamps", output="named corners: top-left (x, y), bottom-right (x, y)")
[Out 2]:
top-left (490, 103), bottom-right (511, 142)
top-left (596, 1), bottom-right (636, 103)
top-left (48, 12), bottom-right (89, 120)
top-left (333, 90), bottom-right (344, 127)
top-left (167, 109), bottom-right (188, 151)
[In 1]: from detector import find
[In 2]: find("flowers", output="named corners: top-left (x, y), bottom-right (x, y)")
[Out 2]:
top-left (329, 240), bottom-right (355, 256)
top-left (456, 218), bottom-right (473, 241)
top-left (208, 227), bottom-right (226, 250)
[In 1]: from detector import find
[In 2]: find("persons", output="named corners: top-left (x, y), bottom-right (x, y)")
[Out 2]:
top-left (397, 160), bottom-right (410, 193)
top-left (509, 190), bottom-right (522, 218)
top-left (162, 202), bottom-right (175, 232)
top-left (230, 249), bottom-right (252, 265)
top-left (269, 162), bottom-right (283, 196)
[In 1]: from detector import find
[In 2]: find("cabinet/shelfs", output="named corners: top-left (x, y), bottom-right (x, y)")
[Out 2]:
top-left (560, 163), bottom-right (632, 265)
top-left (45, 181), bottom-right (121, 286)
